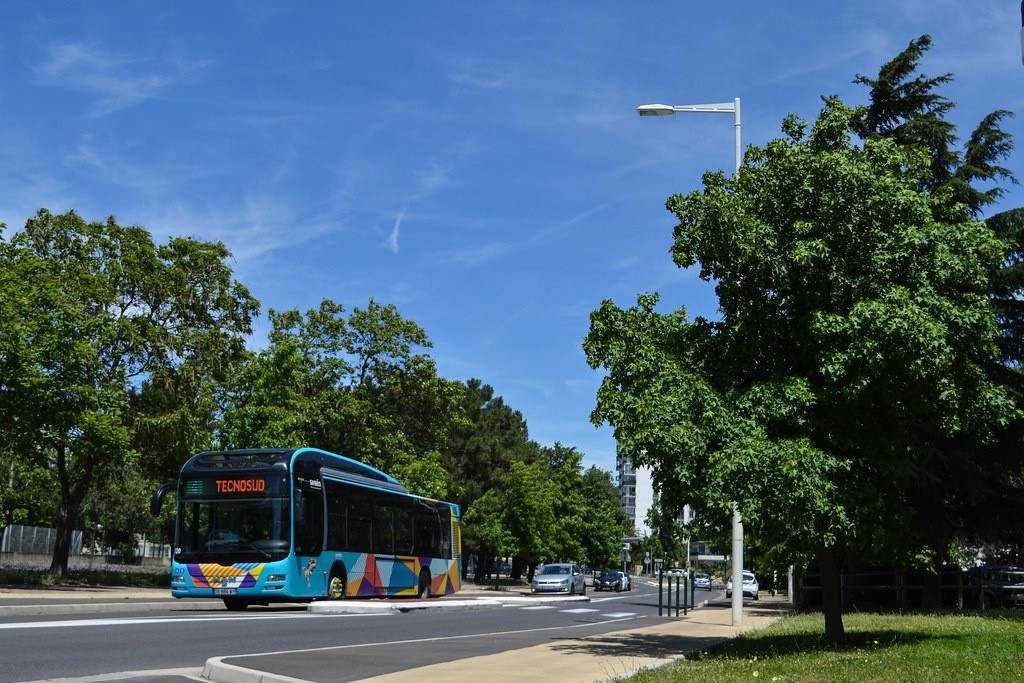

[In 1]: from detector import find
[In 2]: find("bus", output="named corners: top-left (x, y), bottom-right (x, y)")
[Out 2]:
top-left (149, 446), bottom-right (462, 612)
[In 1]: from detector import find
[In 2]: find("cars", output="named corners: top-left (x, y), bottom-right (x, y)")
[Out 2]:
top-left (530, 563), bottom-right (586, 596)
top-left (592, 570), bottom-right (632, 592)
top-left (725, 569), bottom-right (761, 599)
top-left (662, 569), bottom-right (685, 578)
top-left (694, 573), bottom-right (713, 591)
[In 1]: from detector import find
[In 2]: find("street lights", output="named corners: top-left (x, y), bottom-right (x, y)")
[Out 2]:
top-left (635, 95), bottom-right (746, 626)
